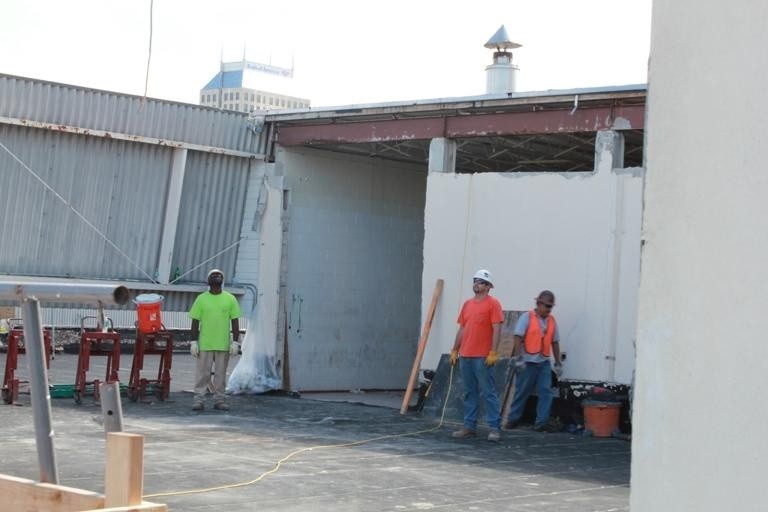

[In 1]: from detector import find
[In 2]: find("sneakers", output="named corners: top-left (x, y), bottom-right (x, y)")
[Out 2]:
top-left (452, 430), bottom-right (479, 439)
top-left (502, 422), bottom-right (517, 430)
top-left (488, 431), bottom-right (500, 441)
top-left (192, 403), bottom-right (203, 410)
top-left (535, 424), bottom-right (556, 433)
top-left (214, 402), bottom-right (229, 411)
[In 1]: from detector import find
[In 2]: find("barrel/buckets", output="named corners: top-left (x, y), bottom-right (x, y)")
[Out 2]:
top-left (132, 293), bottom-right (164, 333)
top-left (579, 399), bottom-right (623, 438)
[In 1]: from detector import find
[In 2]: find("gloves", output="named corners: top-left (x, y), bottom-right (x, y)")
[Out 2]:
top-left (552, 361), bottom-right (563, 377)
top-left (189, 340), bottom-right (199, 357)
top-left (513, 356), bottom-right (525, 370)
top-left (449, 351), bottom-right (459, 364)
top-left (484, 350), bottom-right (498, 368)
top-left (229, 341), bottom-right (240, 355)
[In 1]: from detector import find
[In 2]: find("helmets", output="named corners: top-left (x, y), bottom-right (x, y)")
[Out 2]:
top-left (208, 269), bottom-right (223, 278)
top-left (472, 269), bottom-right (496, 288)
top-left (535, 290), bottom-right (555, 306)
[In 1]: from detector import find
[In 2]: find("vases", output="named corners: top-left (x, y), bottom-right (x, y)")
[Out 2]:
top-left (584, 400), bottom-right (622, 437)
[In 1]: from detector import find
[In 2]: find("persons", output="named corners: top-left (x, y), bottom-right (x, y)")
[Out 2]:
top-left (187, 269), bottom-right (242, 412)
top-left (449, 268), bottom-right (507, 442)
top-left (503, 291), bottom-right (565, 435)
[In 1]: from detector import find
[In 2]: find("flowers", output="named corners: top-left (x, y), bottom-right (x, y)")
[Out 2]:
top-left (583, 384), bottom-right (616, 401)
